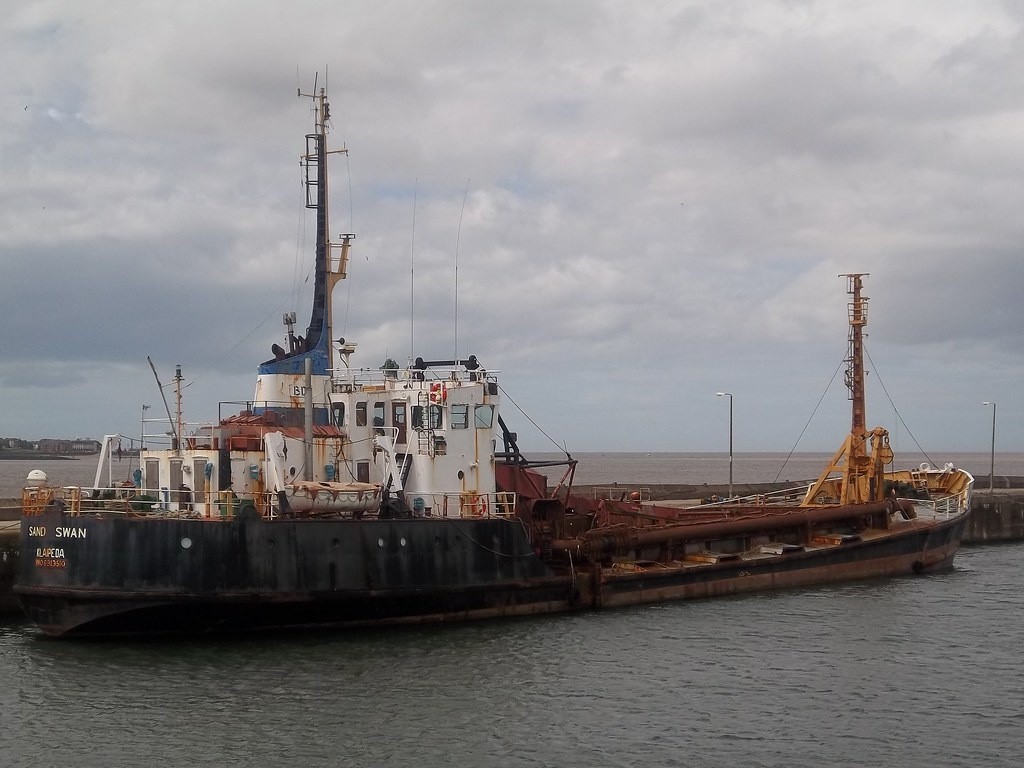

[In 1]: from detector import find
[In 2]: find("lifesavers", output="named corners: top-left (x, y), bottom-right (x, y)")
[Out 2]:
top-left (432, 383), bottom-right (447, 403)
top-left (472, 497), bottom-right (487, 515)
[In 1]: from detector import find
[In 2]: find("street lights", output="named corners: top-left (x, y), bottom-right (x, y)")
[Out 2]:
top-left (717, 393), bottom-right (734, 498)
top-left (982, 401), bottom-right (997, 493)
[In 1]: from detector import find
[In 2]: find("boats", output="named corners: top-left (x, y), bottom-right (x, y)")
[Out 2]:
top-left (277, 481), bottom-right (382, 517)
top-left (11, 62), bottom-right (976, 642)
